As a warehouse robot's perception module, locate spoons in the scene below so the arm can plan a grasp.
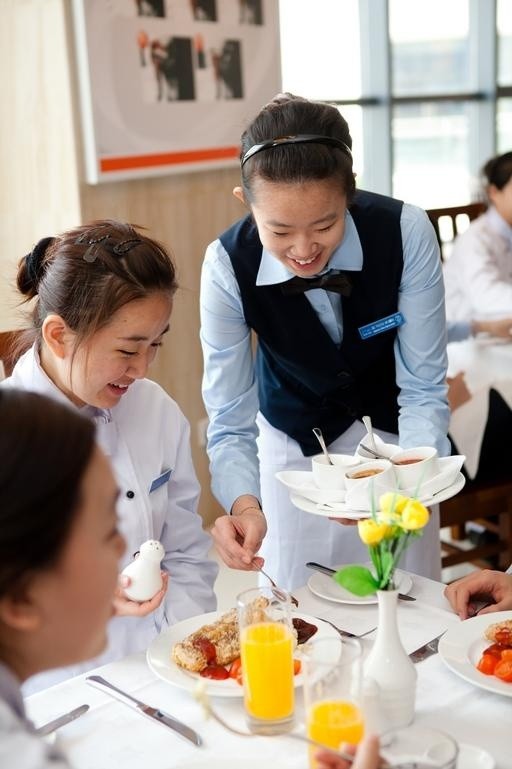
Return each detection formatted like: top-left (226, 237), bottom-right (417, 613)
top-left (250, 561), bottom-right (298, 608)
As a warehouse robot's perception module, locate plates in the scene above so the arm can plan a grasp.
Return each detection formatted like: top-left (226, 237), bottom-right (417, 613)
top-left (146, 610), bottom-right (342, 696)
top-left (290, 471), bottom-right (466, 519)
top-left (438, 611), bottom-right (512, 698)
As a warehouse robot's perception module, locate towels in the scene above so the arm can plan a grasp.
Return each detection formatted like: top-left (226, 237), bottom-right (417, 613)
top-left (295, 580), bottom-right (461, 657)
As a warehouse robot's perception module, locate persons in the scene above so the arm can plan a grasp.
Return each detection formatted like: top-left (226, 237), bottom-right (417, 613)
top-left (0, 387), bottom-right (393, 769)
top-left (199, 92), bottom-right (451, 596)
top-left (442, 152), bottom-right (512, 338)
top-left (1, 218), bottom-right (219, 699)
top-left (444, 564), bottom-right (512, 621)
top-left (445, 318), bottom-right (512, 413)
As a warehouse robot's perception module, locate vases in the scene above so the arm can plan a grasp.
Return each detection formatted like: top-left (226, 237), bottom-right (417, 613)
top-left (352, 591), bottom-right (414, 739)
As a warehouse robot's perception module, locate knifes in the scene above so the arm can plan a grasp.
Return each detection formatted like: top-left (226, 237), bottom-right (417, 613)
top-left (407, 603), bottom-right (492, 663)
top-left (85, 675), bottom-right (201, 747)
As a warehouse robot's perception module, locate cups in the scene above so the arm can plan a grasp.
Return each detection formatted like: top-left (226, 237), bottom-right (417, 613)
top-left (235, 586), bottom-right (295, 735)
top-left (311, 431), bottom-right (438, 508)
top-left (378, 728), bottom-right (458, 769)
top-left (301, 635), bottom-right (365, 769)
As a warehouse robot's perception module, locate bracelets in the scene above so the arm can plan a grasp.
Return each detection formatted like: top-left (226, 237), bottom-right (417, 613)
top-left (238, 506), bottom-right (263, 516)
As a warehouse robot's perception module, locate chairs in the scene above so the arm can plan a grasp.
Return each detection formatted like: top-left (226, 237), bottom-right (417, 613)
top-left (440, 479), bottom-right (510, 574)
top-left (428, 201), bottom-right (488, 266)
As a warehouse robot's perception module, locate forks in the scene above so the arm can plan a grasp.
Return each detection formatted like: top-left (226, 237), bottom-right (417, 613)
top-left (315, 614), bottom-right (377, 638)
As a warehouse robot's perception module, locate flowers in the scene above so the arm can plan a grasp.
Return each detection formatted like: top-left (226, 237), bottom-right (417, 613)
top-left (334, 433), bottom-right (429, 596)
